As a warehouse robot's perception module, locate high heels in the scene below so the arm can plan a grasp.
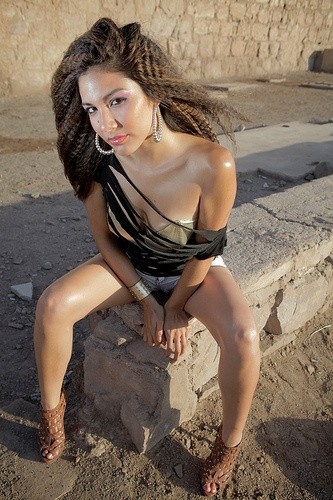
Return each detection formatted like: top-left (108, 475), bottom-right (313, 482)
top-left (37, 393), bottom-right (67, 463)
top-left (200, 422), bottom-right (242, 497)
